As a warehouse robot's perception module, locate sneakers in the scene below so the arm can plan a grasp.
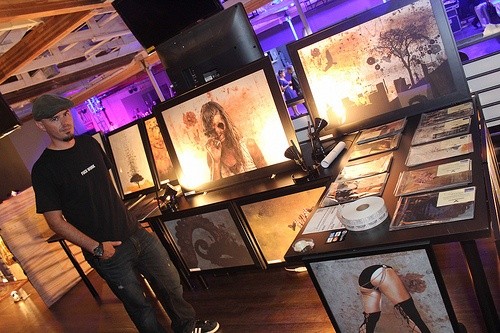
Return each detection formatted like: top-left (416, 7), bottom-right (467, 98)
top-left (189, 320), bottom-right (220, 333)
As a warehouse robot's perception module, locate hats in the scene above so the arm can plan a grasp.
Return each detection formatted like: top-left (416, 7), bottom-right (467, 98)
top-left (31, 95), bottom-right (73, 121)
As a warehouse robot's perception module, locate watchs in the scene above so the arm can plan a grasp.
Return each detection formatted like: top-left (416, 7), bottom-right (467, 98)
top-left (93, 242), bottom-right (104, 257)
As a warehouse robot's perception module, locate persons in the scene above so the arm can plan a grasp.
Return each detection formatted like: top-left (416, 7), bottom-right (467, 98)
top-left (201, 101), bottom-right (267, 182)
top-left (278, 70), bottom-right (300, 115)
top-left (358, 264), bottom-right (431, 333)
top-left (287, 65), bottom-right (308, 111)
top-left (31, 94), bottom-right (220, 333)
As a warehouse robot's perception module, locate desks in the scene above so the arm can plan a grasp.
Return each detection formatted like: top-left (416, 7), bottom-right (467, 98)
top-left (45, 93), bottom-right (500, 333)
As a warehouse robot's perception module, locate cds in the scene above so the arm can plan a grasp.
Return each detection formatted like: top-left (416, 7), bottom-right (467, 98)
top-left (338, 197), bottom-right (388, 231)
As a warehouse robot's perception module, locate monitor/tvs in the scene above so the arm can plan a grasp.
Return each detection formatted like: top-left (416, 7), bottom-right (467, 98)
top-left (156, 1), bottom-right (265, 94)
top-left (150, 54), bottom-right (304, 197)
top-left (285, 0), bottom-right (473, 139)
top-left (0, 91), bottom-right (23, 139)
top-left (102, 119), bottom-right (161, 200)
top-left (110, 0), bottom-right (224, 54)
